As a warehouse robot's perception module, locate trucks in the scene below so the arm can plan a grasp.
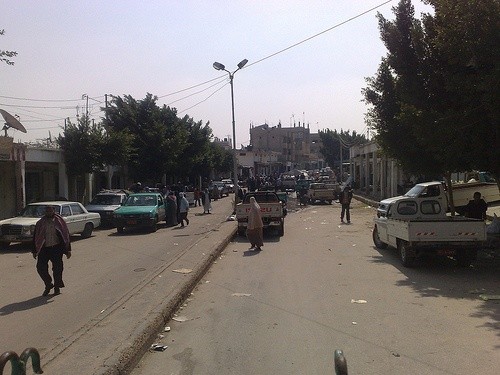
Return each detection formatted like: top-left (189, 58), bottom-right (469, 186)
top-left (296, 179), bottom-right (315, 198)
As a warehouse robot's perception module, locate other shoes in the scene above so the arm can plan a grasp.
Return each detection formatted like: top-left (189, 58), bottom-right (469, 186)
top-left (180, 224), bottom-right (184, 228)
top-left (347, 219), bottom-right (350, 223)
top-left (187, 219), bottom-right (189, 225)
top-left (341, 218), bottom-right (344, 222)
top-left (53, 288), bottom-right (60, 296)
top-left (42, 282), bottom-right (53, 296)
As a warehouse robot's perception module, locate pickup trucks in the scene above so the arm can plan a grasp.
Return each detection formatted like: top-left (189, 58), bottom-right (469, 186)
top-left (206, 181), bottom-right (230, 199)
top-left (305, 183), bottom-right (333, 204)
top-left (373, 196), bottom-right (487, 269)
top-left (114, 193), bottom-right (166, 233)
top-left (377, 180), bottom-right (500, 219)
top-left (235, 192), bottom-right (285, 237)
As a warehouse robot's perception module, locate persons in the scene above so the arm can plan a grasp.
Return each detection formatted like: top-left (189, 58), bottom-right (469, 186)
top-left (464, 192), bottom-right (487, 219)
top-left (128, 172), bottom-right (353, 228)
top-left (247, 197), bottom-right (264, 252)
top-left (32, 205), bottom-right (71, 296)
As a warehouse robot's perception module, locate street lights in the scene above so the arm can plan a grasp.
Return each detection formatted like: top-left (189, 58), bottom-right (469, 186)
top-left (213, 59), bottom-right (248, 213)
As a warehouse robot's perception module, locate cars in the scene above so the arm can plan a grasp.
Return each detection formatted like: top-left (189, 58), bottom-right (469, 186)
top-left (279, 166), bottom-right (337, 196)
top-left (83, 192), bottom-right (126, 227)
top-left (221, 179), bottom-right (235, 194)
top-left (0, 201), bottom-right (101, 251)
